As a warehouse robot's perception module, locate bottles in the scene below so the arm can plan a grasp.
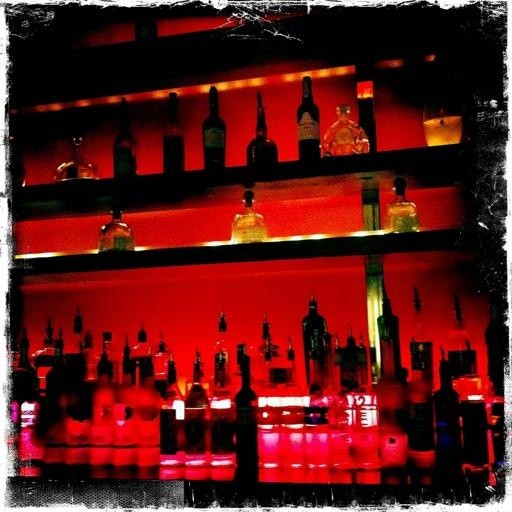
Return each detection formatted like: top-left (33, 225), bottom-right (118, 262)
top-left (55, 133), bottom-right (94, 181)
top-left (112, 98), bottom-right (138, 177)
top-left (17, 292), bottom-right (487, 469)
top-left (383, 177), bottom-right (419, 232)
top-left (201, 87), bottom-right (226, 166)
top-left (100, 202), bottom-right (132, 255)
top-left (294, 77), bottom-right (324, 160)
top-left (320, 104), bottom-right (371, 154)
top-left (246, 93), bottom-right (279, 165)
top-left (162, 91), bottom-right (184, 171)
top-left (231, 181), bottom-right (268, 243)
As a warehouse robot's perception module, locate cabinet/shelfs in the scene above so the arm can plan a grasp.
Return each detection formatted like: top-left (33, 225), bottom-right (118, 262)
top-left (12, 143), bottom-right (466, 274)
